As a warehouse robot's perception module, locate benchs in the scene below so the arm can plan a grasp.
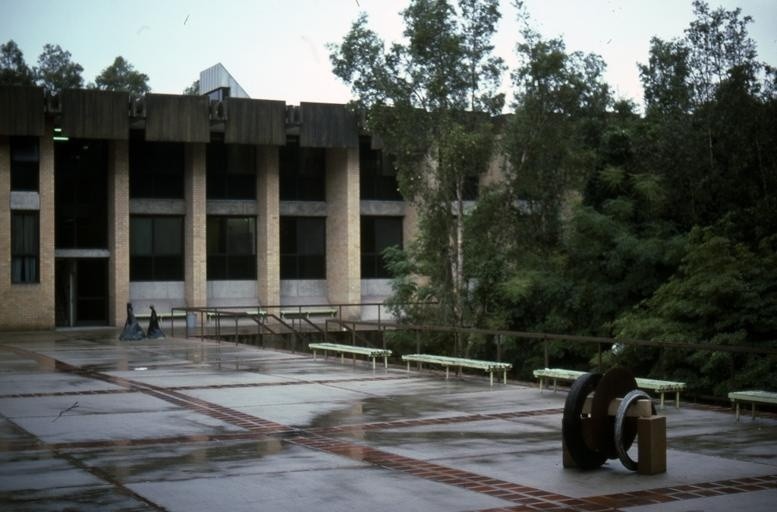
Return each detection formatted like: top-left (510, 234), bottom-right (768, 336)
top-left (727, 389), bottom-right (776, 420)
top-left (401, 352), bottom-right (513, 385)
top-left (133, 311), bottom-right (186, 325)
top-left (533, 367), bottom-right (687, 408)
top-left (309, 342), bottom-right (394, 370)
top-left (206, 309), bottom-right (338, 325)
